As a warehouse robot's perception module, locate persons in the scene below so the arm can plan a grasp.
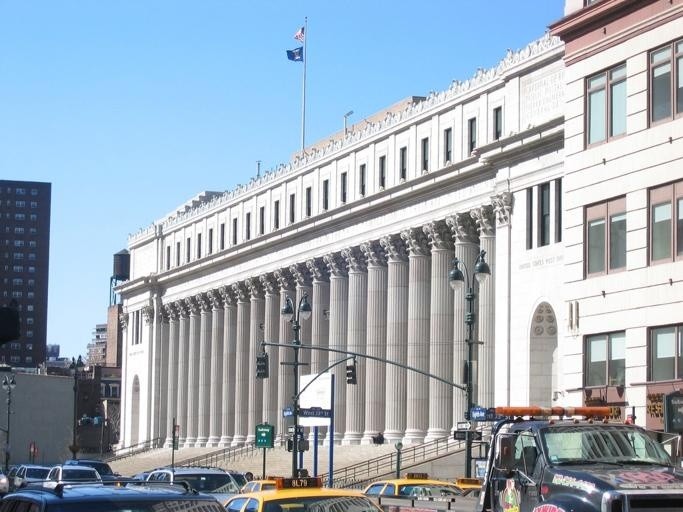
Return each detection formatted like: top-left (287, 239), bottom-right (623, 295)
top-left (245, 472), bottom-right (253, 481)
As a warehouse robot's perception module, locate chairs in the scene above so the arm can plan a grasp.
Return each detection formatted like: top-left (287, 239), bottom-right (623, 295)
top-left (517, 445), bottom-right (540, 476)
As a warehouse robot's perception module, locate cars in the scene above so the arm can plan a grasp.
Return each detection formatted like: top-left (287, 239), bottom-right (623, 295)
top-left (0, 458), bottom-right (485, 511)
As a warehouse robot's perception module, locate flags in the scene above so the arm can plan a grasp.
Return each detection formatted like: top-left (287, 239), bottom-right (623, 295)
top-left (286, 25), bottom-right (304, 63)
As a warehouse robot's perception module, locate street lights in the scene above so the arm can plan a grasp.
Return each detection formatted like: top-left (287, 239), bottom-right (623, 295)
top-left (0, 375), bottom-right (16, 467)
top-left (447, 251), bottom-right (490, 479)
top-left (279, 293), bottom-right (312, 480)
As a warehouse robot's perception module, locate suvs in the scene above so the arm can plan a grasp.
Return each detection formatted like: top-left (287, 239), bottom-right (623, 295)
top-left (478, 404), bottom-right (683, 511)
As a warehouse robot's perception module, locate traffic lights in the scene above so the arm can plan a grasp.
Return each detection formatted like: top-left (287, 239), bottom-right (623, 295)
top-left (255, 354), bottom-right (268, 378)
top-left (344, 365), bottom-right (355, 383)
